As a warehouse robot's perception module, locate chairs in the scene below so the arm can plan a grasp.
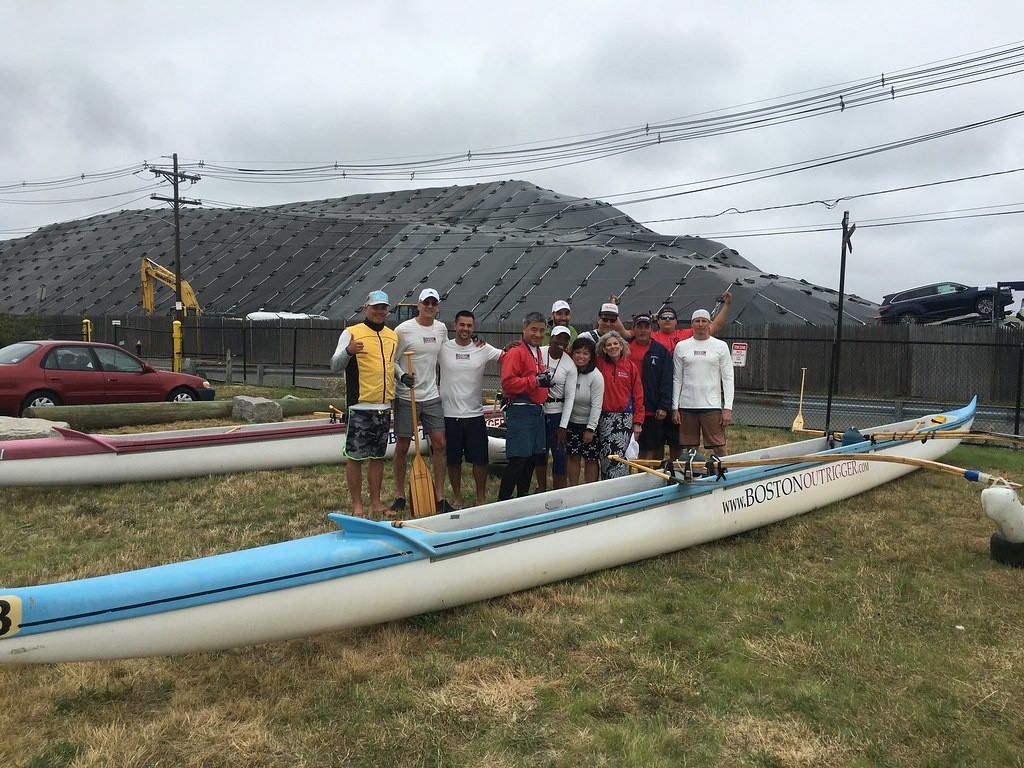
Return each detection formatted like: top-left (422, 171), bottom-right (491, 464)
top-left (59, 353), bottom-right (77, 369)
top-left (74, 356), bottom-right (92, 370)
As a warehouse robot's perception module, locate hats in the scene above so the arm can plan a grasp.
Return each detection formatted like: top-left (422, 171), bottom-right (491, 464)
top-left (691, 309), bottom-right (712, 326)
top-left (625, 432), bottom-right (639, 461)
top-left (598, 303), bottom-right (619, 316)
top-left (364, 291), bottom-right (391, 308)
top-left (658, 306), bottom-right (677, 328)
top-left (418, 288), bottom-right (440, 302)
top-left (632, 316), bottom-right (652, 328)
top-left (551, 325), bottom-right (571, 338)
top-left (550, 300), bottom-right (571, 313)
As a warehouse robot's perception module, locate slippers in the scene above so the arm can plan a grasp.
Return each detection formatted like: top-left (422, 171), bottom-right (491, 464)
top-left (453, 499), bottom-right (464, 509)
top-left (368, 507), bottom-right (396, 517)
top-left (352, 509), bottom-right (369, 520)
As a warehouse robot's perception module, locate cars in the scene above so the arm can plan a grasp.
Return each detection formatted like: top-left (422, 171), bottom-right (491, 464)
top-left (0, 340), bottom-right (216, 419)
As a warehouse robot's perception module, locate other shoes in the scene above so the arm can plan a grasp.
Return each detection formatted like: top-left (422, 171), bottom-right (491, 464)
top-left (436, 498), bottom-right (455, 513)
top-left (391, 497), bottom-right (406, 512)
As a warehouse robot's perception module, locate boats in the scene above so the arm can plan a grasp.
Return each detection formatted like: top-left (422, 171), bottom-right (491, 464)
top-left (0, 405), bottom-right (520, 487)
top-left (0, 395), bottom-right (979, 667)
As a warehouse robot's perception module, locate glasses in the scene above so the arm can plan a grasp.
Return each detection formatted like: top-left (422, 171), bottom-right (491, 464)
top-left (660, 314), bottom-right (675, 320)
top-left (419, 300), bottom-right (438, 306)
top-left (601, 318), bottom-right (615, 322)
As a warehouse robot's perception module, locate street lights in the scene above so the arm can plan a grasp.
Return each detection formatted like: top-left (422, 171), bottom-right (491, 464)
top-left (134, 214), bottom-right (182, 357)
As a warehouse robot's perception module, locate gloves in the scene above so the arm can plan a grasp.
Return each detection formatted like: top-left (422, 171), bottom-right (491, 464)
top-left (535, 369), bottom-right (551, 387)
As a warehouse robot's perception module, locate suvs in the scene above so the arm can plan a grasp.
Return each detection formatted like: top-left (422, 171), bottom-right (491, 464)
top-left (879, 282), bottom-right (1014, 326)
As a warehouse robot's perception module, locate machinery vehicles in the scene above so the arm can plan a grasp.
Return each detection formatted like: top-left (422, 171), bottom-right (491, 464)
top-left (140, 253), bottom-right (202, 317)
top-left (346, 303), bottom-right (441, 321)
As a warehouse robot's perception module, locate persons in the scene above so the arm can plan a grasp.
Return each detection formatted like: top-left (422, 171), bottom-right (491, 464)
top-left (504, 292), bottom-right (735, 490)
top-left (330, 290), bottom-right (415, 521)
top-left (437, 310), bottom-right (504, 510)
top-left (391, 288), bottom-right (486, 513)
top-left (497, 312), bottom-right (557, 503)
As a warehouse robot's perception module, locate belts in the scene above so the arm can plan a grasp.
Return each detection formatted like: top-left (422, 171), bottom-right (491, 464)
top-left (512, 398), bottom-right (533, 403)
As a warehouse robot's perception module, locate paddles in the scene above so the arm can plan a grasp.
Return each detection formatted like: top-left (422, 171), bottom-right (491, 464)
top-left (794, 429), bottom-right (1024, 444)
top-left (403, 351), bottom-right (437, 518)
top-left (608, 454), bottom-right (686, 485)
top-left (912, 420), bottom-right (925, 430)
top-left (630, 453), bottom-right (1024, 491)
top-left (792, 368), bottom-right (807, 430)
top-left (225, 426), bottom-right (241, 433)
